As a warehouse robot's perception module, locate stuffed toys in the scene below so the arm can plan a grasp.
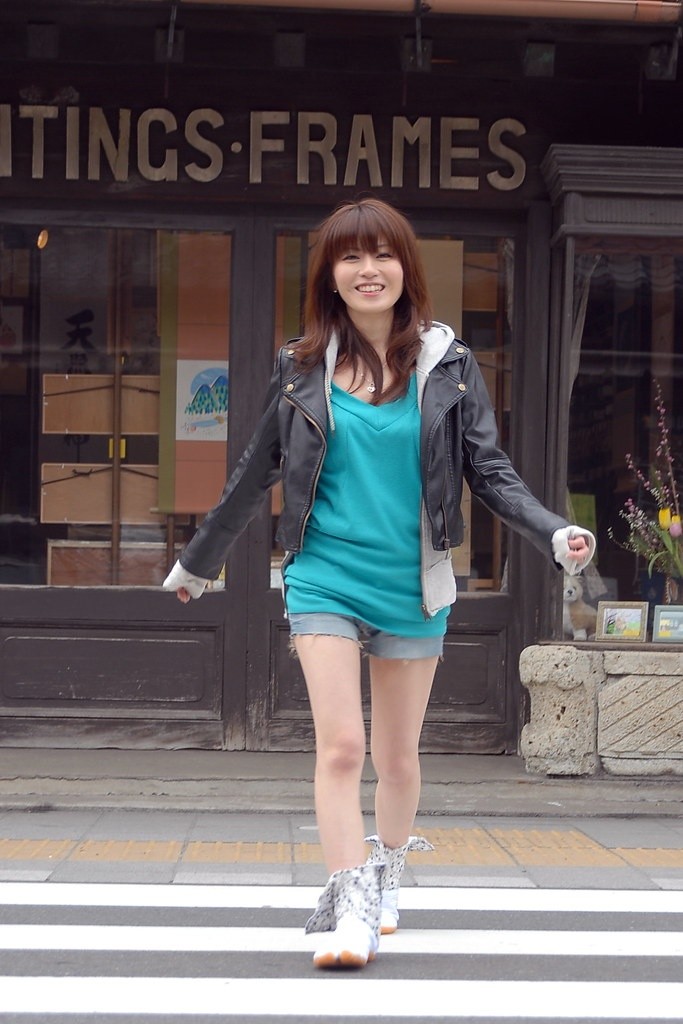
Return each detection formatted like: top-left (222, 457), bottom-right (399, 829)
top-left (564, 574), bottom-right (597, 640)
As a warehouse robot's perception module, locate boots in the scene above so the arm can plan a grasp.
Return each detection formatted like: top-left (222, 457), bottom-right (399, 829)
top-left (305, 861), bottom-right (386, 969)
top-left (364, 832), bottom-right (435, 936)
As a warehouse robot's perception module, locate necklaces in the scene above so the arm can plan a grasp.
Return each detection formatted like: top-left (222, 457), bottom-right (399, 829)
top-left (343, 351), bottom-right (389, 394)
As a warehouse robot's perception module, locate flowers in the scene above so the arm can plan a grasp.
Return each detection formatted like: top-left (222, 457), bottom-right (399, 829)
top-left (607, 375), bottom-right (683, 605)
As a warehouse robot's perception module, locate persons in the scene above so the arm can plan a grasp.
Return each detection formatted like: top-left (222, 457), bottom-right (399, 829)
top-left (161, 199), bottom-right (599, 967)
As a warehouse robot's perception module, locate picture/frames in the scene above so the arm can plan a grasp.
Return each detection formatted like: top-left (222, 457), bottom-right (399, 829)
top-left (652, 605), bottom-right (683, 644)
top-left (596, 601), bottom-right (649, 643)
